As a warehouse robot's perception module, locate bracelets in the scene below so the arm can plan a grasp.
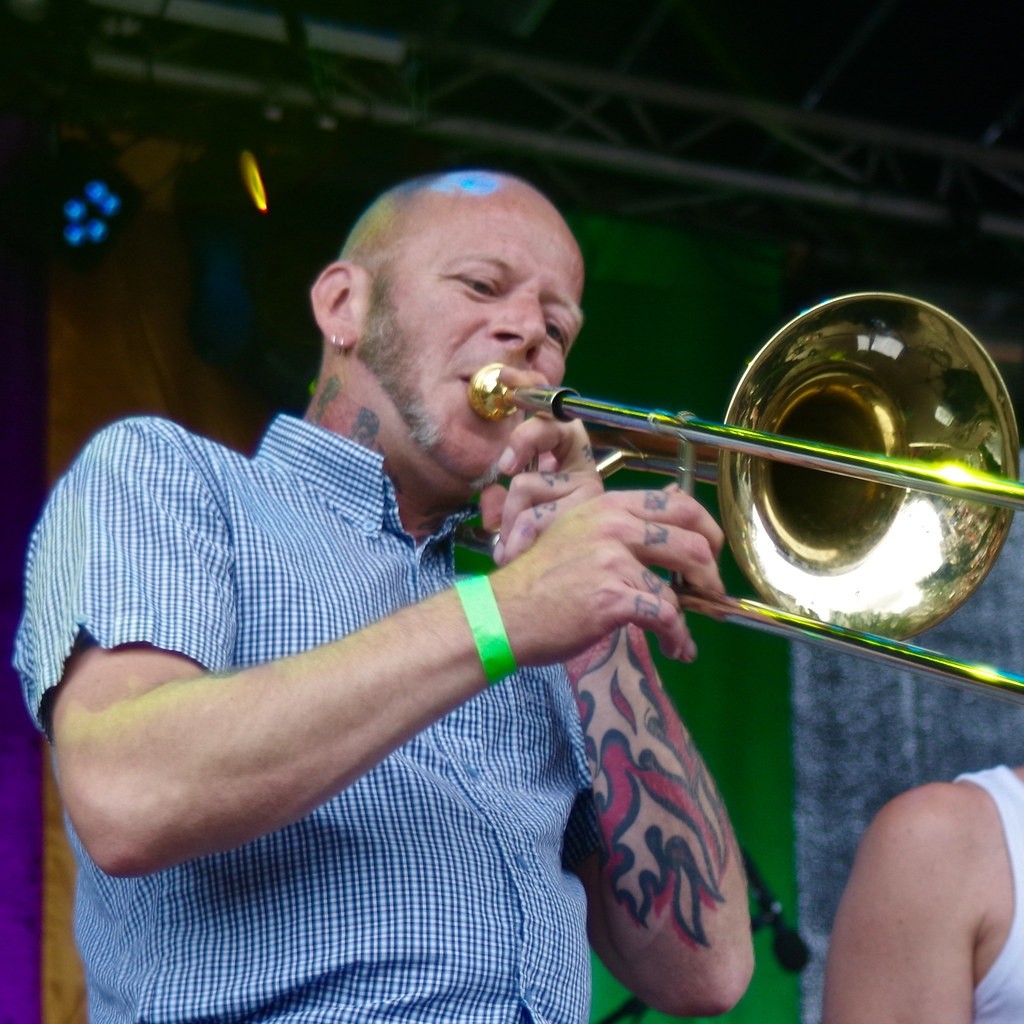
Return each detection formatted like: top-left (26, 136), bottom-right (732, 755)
top-left (453, 572), bottom-right (516, 683)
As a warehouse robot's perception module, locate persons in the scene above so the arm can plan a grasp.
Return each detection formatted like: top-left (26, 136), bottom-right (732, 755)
top-left (14, 172), bottom-right (755, 1024)
top-left (822, 764), bottom-right (1024, 1023)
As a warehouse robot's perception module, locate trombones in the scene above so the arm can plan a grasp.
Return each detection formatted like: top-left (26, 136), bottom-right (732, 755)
top-left (467, 288), bottom-right (1024, 695)
